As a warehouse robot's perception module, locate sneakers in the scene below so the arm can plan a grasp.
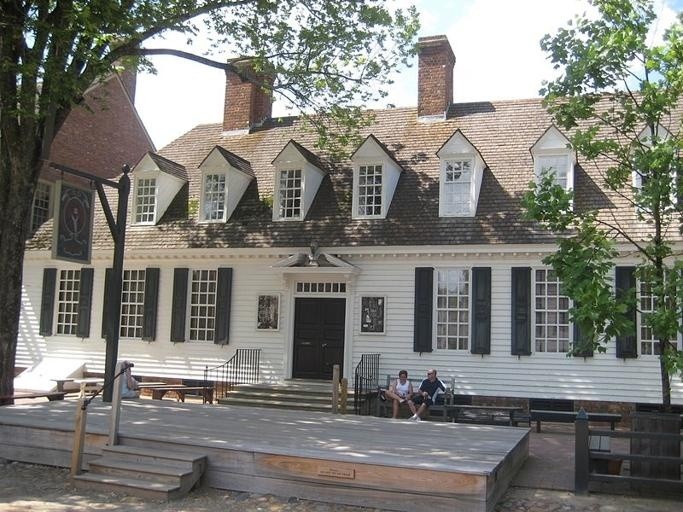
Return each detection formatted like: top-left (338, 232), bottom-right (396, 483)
top-left (408, 417), bottom-right (421, 421)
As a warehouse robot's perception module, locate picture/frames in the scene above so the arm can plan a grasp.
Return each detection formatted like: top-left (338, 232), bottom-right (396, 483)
top-left (255, 294), bottom-right (279, 332)
top-left (361, 295), bottom-right (386, 336)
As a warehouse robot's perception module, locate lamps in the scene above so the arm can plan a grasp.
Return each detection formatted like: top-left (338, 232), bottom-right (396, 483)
top-left (308, 240), bottom-right (321, 265)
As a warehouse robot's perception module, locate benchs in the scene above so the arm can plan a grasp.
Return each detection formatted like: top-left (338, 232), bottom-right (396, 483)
top-left (376, 374), bottom-right (622, 472)
top-left (0, 378), bottom-right (216, 404)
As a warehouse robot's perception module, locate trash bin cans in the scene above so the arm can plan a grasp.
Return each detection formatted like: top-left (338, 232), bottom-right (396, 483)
top-left (589, 449), bottom-right (623, 475)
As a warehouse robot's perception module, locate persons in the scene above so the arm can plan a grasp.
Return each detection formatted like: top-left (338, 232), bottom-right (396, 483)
top-left (385, 370), bottom-right (414, 418)
top-left (122, 361), bottom-right (141, 399)
top-left (408, 369), bottom-right (448, 420)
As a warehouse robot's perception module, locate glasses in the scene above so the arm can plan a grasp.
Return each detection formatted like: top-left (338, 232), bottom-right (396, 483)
top-left (427, 373), bottom-right (433, 375)
